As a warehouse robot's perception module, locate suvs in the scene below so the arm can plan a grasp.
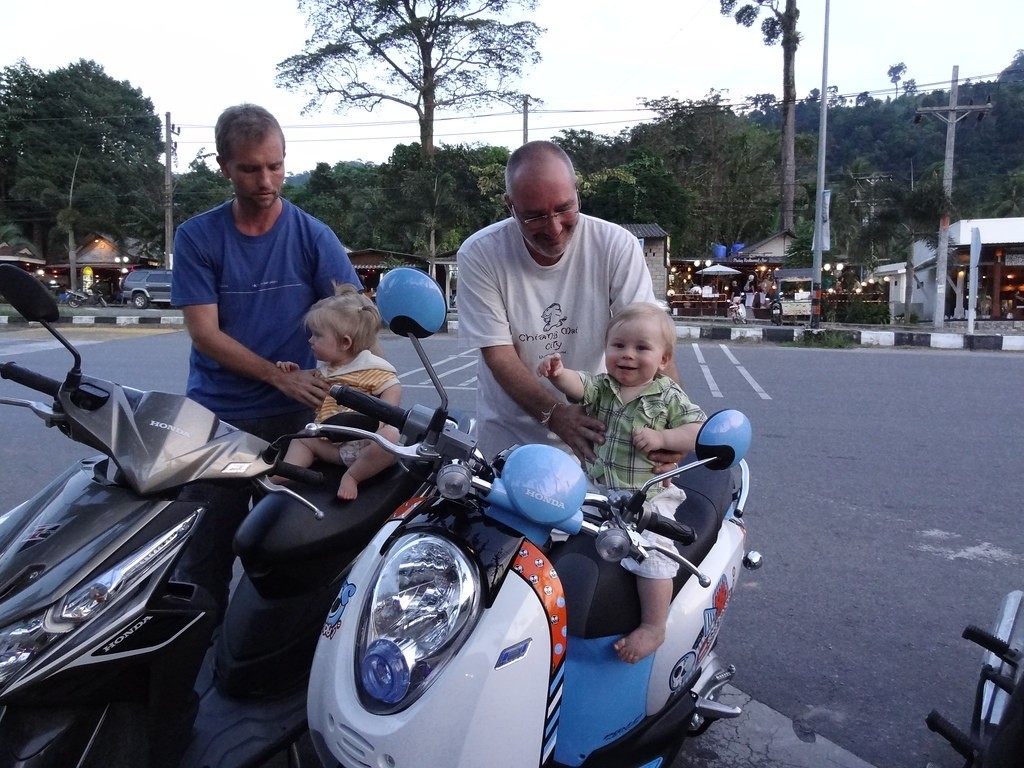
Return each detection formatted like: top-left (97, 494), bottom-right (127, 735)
top-left (120, 268), bottom-right (173, 309)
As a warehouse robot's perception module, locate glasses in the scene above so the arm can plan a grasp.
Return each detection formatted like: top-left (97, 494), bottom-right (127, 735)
top-left (512, 191), bottom-right (581, 226)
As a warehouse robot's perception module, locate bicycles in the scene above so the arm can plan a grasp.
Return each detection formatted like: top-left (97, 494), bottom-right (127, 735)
top-left (729, 303), bottom-right (747, 325)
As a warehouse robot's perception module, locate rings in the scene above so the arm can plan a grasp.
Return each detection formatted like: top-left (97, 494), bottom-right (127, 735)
top-left (674, 463), bottom-right (679, 468)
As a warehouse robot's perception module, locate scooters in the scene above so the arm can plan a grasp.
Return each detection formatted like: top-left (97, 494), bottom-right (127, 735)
top-left (0, 258), bottom-right (456, 768)
top-left (282, 263), bottom-right (763, 767)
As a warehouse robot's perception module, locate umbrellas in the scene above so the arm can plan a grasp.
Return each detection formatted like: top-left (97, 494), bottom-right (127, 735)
top-left (695, 263), bottom-right (742, 293)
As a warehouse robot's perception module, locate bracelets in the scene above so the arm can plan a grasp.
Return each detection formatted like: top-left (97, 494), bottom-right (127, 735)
top-left (540, 402), bottom-right (565, 432)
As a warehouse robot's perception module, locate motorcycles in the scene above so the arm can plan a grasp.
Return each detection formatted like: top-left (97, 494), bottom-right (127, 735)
top-left (764, 287), bottom-right (784, 326)
top-left (64, 274), bottom-right (109, 309)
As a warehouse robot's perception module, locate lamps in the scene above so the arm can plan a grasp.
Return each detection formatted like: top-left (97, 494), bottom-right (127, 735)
top-left (995, 248), bottom-right (1002, 263)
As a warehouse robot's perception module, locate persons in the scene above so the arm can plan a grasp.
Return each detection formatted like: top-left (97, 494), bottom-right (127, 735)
top-left (170, 102), bottom-right (385, 618)
top-left (270, 282), bottom-right (402, 499)
top-left (836, 275), bottom-right (861, 293)
top-left (456, 141), bottom-right (678, 487)
top-left (746, 275), bottom-right (755, 295)
top-left (535, 303), bottom-right (708, 664)
top-left (732, 280), bottom-right (742, 296)
